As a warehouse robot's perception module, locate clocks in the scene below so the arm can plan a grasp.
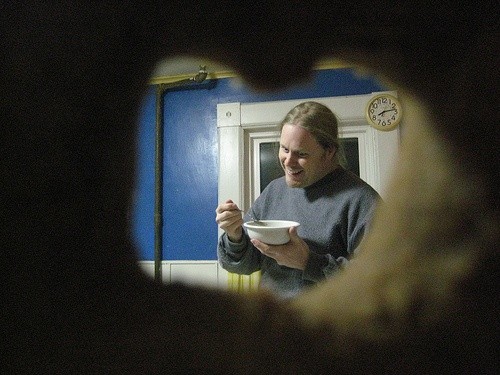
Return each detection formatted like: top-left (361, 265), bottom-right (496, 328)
top-left (365, 94), bottom-right (403, 131)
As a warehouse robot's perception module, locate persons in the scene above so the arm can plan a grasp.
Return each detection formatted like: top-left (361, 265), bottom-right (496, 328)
top-left (215, 101), bottom-right (385, 298)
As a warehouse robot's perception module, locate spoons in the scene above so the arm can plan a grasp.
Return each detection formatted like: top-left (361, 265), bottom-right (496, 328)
top-left (239, 210), bottom-right (268, 226)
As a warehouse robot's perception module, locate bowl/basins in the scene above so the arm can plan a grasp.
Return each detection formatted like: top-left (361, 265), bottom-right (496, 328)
top-left (243, 220), bottom-right (300, 245)
